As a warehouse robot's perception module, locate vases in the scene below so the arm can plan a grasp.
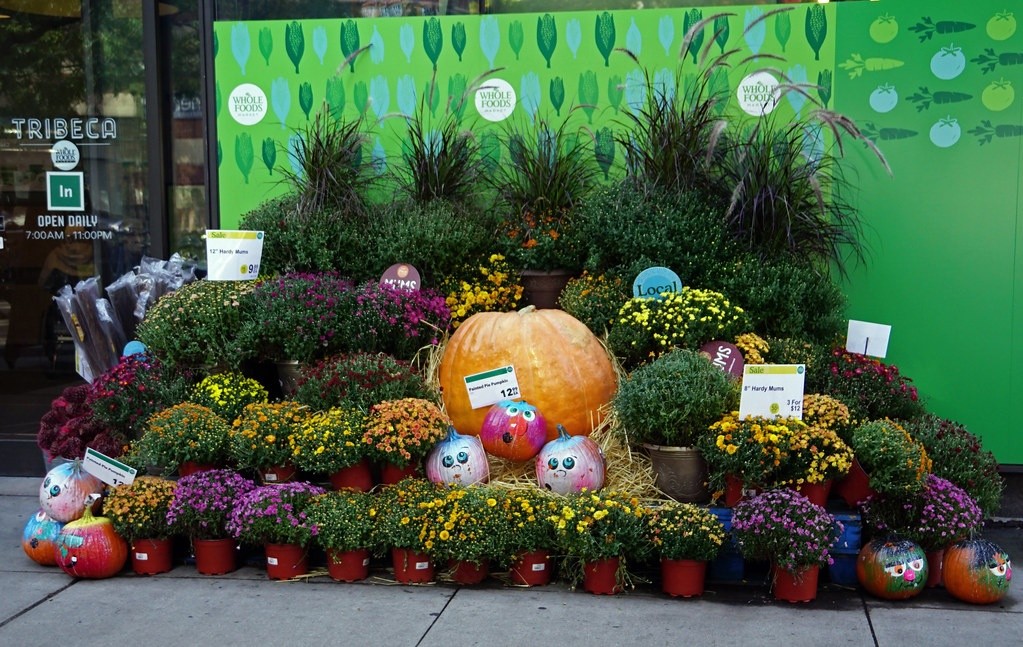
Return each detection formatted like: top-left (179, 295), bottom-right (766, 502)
top-left (380, 460), bottom-right (419, 487)
top-left (799, 477), bottom-right (834, 507)
top-left (660, 556), bottom-right (706, 598)
top-left (643, 443), bottom-right (706, 503)
top-left (584, 556), bottom-right (624, 596)
top-left (180, 460), bottom-right (217, 476)
top-left (838, 456), bottom-right (870, 508)
top-left (445, 556), bottom-right (486, 584)
top-left (724, 474), bottom-right (762, 508)
top-left (130, 537), bottom-right (172, 574)
top-left (327, 546), bottom-right (370, 583)
top-left (509, 549), bottom-right (553, 584)
top-left (773, 563), bottom-right (816, 603)
top-left (518, 269), bottom-right (567, 308)
top-left (264, 540), bottom-right (306, 579)
top-left (191, 536), bottom-right (239, 574)
top-left (394, 548), bottom-right (435, 584)
top-left (925, 549), bottom-right (946, 587)
top-left (273, 359), bottom-right (313, 396)
top-left (263, 462), bottom-right (295, 484)
top-left (330, 459), bottom-right (371, 492)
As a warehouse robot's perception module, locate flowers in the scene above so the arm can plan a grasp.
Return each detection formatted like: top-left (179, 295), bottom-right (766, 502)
top-left (38, 7), bottom-right (1004, 593)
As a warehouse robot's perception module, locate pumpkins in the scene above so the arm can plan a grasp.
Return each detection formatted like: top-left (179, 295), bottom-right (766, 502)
top-left (855, 530), bottom-right (1012, 603)
top-left (437, 304), bottom-right (620, 444)
top-left (425, 399), bottom-right (604, 497)
top-left (23, 455), bottom-right (129, 577)
